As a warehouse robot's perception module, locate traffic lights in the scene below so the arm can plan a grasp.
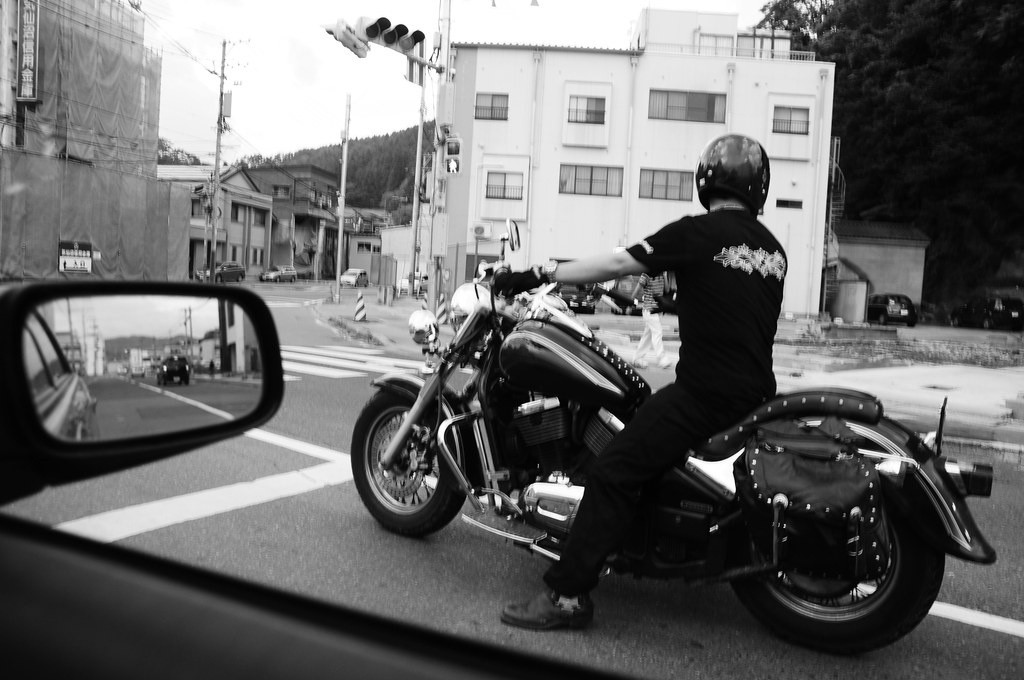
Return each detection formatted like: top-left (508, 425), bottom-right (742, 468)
top-left (443, 138), bottom-right (460, 174)
top-left (353, 13), bottom-right (426, 57)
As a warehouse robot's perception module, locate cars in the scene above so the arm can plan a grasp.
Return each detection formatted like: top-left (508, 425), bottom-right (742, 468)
top-left (156, 355), bottom-right (192, 385)
top-left (259, 264), bottom-right (298, 283)
top-left (868, 291), bottom-right (918, 326)
top-left (948, 293), bottom-right (1024, 331)
top-left (339, 268), bottom-right (368, 288)
top-left (547, 257), bottom-right (599, 314)
top-left (611, 271), bottom-right (663, 316)
top-left (117, 368), bottom-right (130, 377)
top-left (194, 261), bottom-right (246, 282)
top-left (132, 363), bottom-right (146, 378)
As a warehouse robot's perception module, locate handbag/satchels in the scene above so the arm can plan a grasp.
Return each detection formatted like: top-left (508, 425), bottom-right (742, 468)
top-left (654, 292), bottom-right (673, 310)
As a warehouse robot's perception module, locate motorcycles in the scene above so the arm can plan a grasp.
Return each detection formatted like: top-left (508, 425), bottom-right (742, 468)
top-left (348, 214), bottom-right (998, 662)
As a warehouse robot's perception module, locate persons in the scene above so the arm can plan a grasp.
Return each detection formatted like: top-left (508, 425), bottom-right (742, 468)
top-left (495, 133), bottom-right (788, 630)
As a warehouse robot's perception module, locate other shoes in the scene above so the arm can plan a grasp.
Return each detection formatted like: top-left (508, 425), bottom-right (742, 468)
top-left (656, 360), bottom-right (672, 368)
top-left (632, 361), bottom-right (649, 368)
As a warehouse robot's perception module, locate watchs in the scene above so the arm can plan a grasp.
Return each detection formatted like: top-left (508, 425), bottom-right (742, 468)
top-left (545, 260), bottom-right (558, 283)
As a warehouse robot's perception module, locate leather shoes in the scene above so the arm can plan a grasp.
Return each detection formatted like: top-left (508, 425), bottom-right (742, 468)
top-left (498, 586), bottom-right (594, 629)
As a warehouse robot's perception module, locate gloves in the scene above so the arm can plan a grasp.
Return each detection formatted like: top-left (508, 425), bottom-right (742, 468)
top-left (493, 265), bottom-right (551, 305)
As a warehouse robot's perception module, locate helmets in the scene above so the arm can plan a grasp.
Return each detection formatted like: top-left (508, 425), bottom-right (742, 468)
top-left (695, 134), bottom-right (771, 212)
